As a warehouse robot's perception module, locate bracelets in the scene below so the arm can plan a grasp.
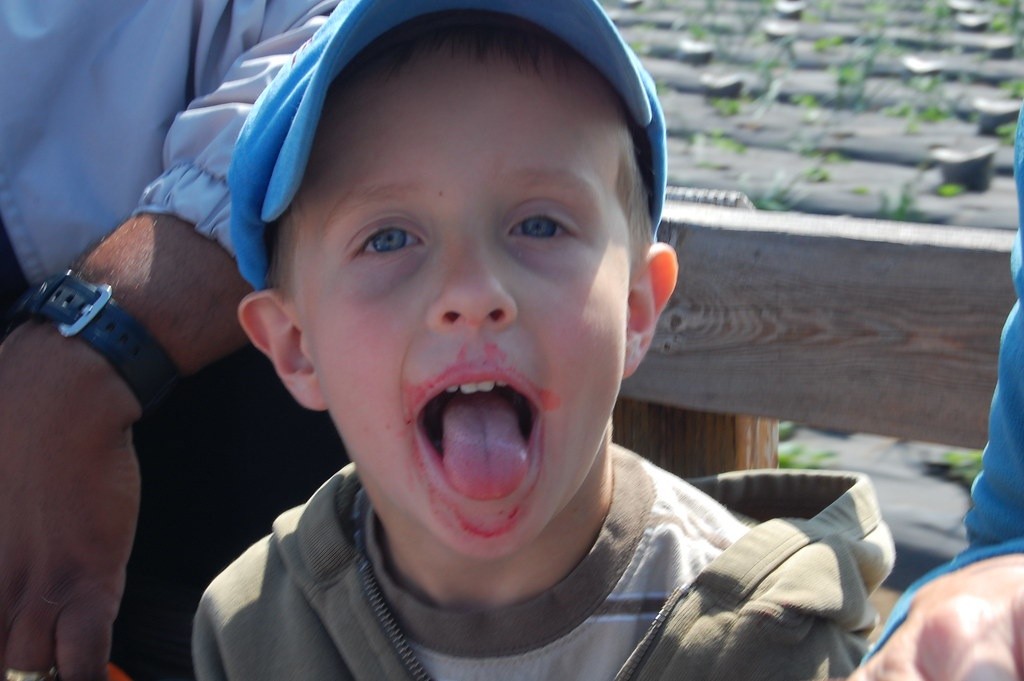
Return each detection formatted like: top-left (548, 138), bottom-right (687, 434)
top-left (12, 270), bottom-right (180, 420)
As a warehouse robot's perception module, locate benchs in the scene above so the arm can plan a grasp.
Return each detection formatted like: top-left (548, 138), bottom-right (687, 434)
top-left (610, 175), bottom-right (1017, 552)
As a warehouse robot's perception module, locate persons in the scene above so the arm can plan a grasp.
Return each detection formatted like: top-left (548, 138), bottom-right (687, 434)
top-left (0, 0), bottom-right (343, 681)
top-left (191, 0), bottom-right (898, 681)
top-left (852, 103), bottom-right (1024, 681)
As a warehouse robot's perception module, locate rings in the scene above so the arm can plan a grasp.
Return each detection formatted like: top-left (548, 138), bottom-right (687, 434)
top-left (4, 663), bottom-right (59, 681)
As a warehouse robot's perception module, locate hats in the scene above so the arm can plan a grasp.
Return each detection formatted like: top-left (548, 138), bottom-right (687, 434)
top-left (229, 0), bottom-right (667, 293)
